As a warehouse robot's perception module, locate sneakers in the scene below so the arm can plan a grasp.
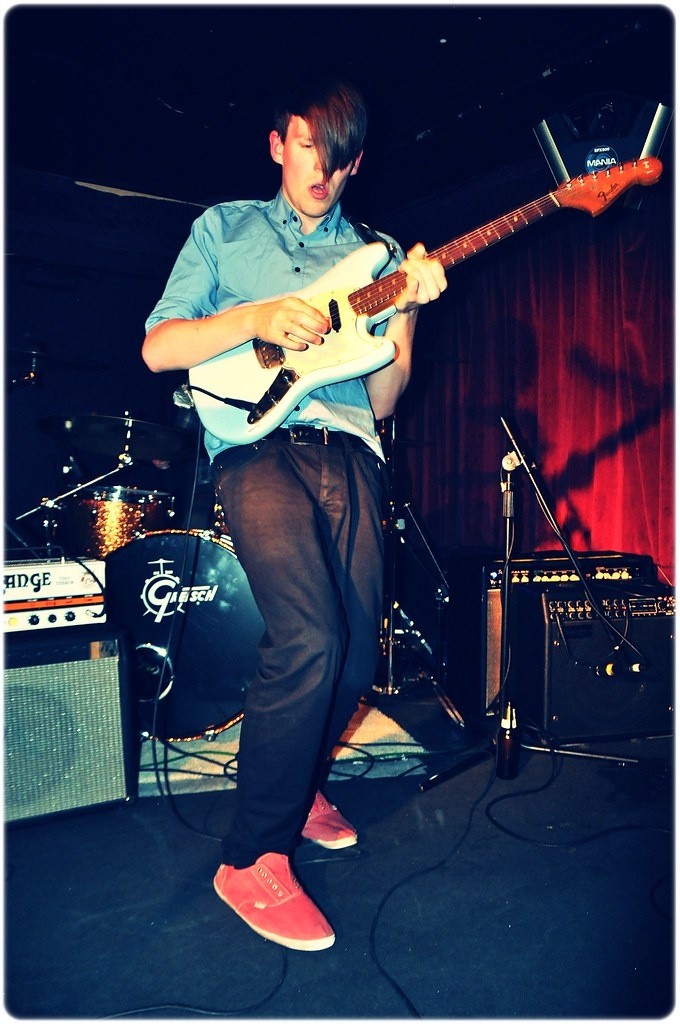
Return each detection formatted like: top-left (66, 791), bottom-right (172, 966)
top-left (301, 789), bottom-right (357, 849)
top-left (214, 853), bottom-right (335, 951)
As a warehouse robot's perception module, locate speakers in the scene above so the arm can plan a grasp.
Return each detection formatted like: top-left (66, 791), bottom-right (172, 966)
top-left (4, 622), bottom-right (136, 822)
top-left (505, 584), bottom-right (675, 748)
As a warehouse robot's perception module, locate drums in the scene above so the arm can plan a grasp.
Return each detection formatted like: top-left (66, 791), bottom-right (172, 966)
top-left (102, 529), bottom-right (266, 740)
top-left (54, 484), bottom-right (175, 560)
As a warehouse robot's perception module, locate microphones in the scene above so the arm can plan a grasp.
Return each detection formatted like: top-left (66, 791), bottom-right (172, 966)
top-left (595, 663), bottom-right (645, 677)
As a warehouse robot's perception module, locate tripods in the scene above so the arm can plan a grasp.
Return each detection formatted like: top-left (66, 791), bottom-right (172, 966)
top-left (418, 415), bottom-right (639, 794)
top-left (372, 414), bottom-right (467, 730)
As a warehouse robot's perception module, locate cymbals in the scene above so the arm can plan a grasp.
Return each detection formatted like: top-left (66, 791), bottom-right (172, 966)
top-left (7, 346), bottom-right (107, 368)
top-left (36, 413), bottom-right (175, 432)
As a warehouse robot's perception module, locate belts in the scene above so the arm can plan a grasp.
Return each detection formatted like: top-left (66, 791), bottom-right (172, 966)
top-left (266, 425), bottom-right (368, 447)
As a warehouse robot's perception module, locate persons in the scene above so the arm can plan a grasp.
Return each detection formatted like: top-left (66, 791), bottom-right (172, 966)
top-left (141, 77), bottom-right (448, 952)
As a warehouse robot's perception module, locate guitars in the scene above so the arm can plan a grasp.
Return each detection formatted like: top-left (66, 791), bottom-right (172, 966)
top-left (187, 156), bottom-right (662, 445)
